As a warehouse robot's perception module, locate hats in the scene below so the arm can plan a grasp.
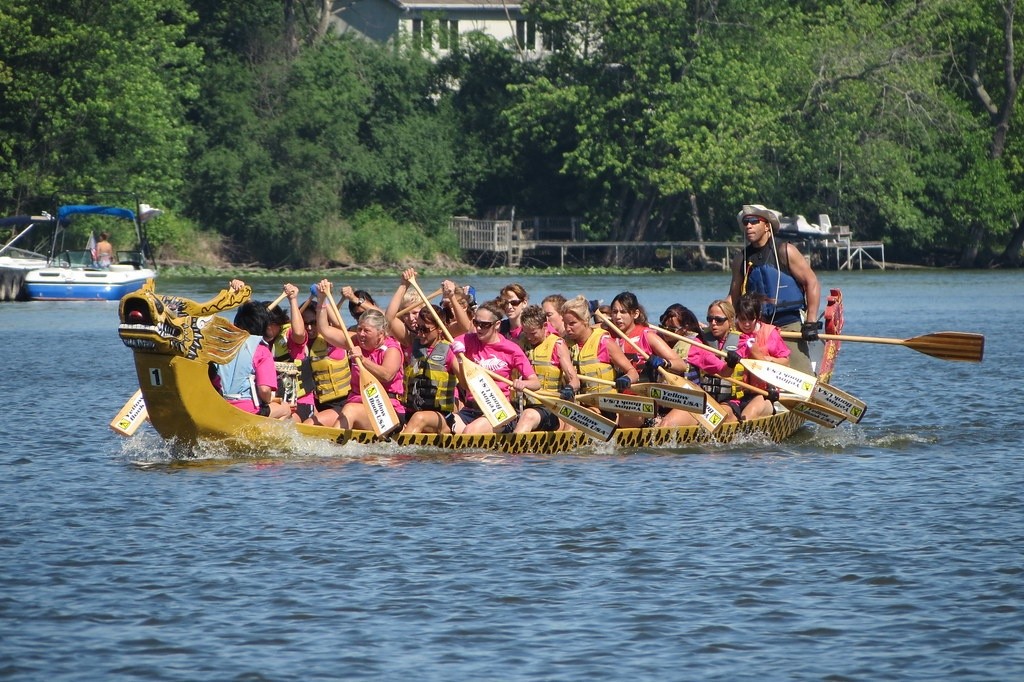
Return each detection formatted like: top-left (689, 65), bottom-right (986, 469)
top-left (736, 205), bottom-right (781, 235)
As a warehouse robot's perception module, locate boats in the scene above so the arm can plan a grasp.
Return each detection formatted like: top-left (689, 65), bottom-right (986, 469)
top-left (21, 189), bottom-right (159, 302)
top-left (0, 214), bottom-right (63, 300)
top-left (118, 279), bottom-right (844, 450)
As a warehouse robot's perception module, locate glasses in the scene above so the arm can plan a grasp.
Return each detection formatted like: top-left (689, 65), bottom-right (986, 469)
top-left (502, 299), bottom-right (523, 306)
top-left (414, 324), bottom-right (438, 333)
top-left (658, 323), bottom-right (684, 334)
top-left (742, 216), bottom-right (760, 226)
top-left (472, 318), bottom-right (498, 329)
top-left (706, 316), bottom-right (731, 323)
top-left (303, 318), bottom-right (316, 326)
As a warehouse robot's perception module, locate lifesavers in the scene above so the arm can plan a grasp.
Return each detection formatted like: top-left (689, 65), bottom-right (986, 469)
top-left (0, 271), bottom-right (25, 301)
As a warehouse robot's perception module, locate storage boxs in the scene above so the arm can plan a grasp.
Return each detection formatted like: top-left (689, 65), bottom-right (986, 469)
top-left (834, 225), bottom-right (851, 235)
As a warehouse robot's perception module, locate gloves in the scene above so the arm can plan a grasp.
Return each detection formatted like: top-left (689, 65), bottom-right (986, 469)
top-left (612, 374), bottom-right (631, 393)
top-left (647, 353), bottom-right (668, 369)
top-left (725, 350), bottom-right (741, 368)
top-left (449, 341), bottom-right (465, 354)
top-left (763, 385), bottom-right (779, 403)
top-left (800, 321), bottom-right (819, 341)
top-left (559, 384), bottom-right (575, 401)
top-left (588, 300), bottom-right (600, 317)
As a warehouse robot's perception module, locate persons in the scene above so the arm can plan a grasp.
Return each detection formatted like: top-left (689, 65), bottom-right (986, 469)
top-left (207, 267), bottom-right (790, 436)
top-left (96, 232), bottom-right (113, 268)
top-left (726, 204), bottom-right (823, 379)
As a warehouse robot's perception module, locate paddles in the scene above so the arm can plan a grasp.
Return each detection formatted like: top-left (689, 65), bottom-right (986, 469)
top-left (714, 372), bottom-right (848, 430)
top-left (561, 370), bottom-right (708, 415)
top-left (486, 368), bottom-right (618, 443)
top-left (646, 322), bottom-right (818, 399)
top-left (407, 273), bottom-right (519, 430)
top-left (335, 295), bottom-right (346, 310)
top-left (533, 388), bottom-right (658, 420)
top-left (701, 323), bottom-right (985, 364)
top-left (595, 307), bottom-right (728, 435)
top-left (298, 291), bottom-right (316, 313)
top-left (396, 282), bottom-right (449, 318)
top-left (321, 282), bottom-right (401, 441)
top-left (812, 380), bottom-right (869, 425)
top-left (108, 284), bottom-right (294, 438)
